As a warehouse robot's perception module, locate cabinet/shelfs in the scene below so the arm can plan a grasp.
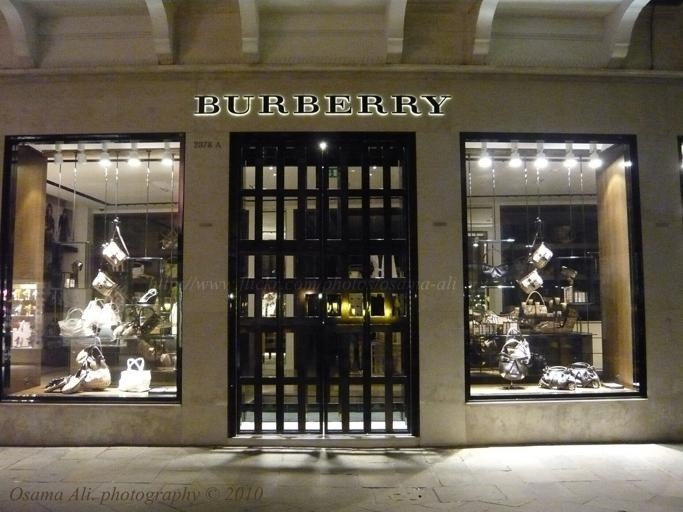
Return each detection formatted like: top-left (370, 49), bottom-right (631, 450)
top-left (10, 279), bottom-right (42, 393)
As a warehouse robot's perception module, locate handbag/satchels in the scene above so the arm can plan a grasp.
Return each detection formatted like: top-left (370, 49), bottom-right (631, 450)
top-left (479, 291), bottom-right (576, 336)
top-left (90, 271), bottom-right (116, 298)
top-left (43, 336), bottom-right (151, 393)
top-left (102, 224), bottom-right (130, 268)
top-left (497, 328), bottom-right (600, 390)
top-left (131, 262), bottom-right (144, 279)
top-left (480, 263), bottom-right (509, 286)
top-left (161, 232), bottom-right (177, 251)
top-left (515, 268), bottom-right (544, 295)
top-left (527, 232), bottom-right (553, 270)
top-left (56, 297), bottom-right (159, 336)
top-left (164, 257), bottom-right (177, 278)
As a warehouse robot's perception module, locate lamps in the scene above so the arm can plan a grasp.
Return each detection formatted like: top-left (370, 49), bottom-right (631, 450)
top-left (54, 139), bottom-right (173, 166)
top-left (477, 139), bottom-right (603, 169)
